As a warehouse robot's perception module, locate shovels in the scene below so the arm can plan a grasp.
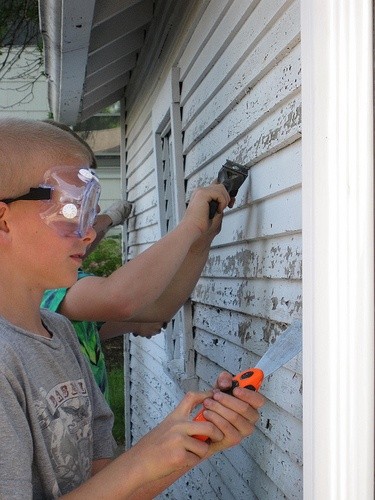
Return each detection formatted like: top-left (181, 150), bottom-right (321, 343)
top-left (185, 316), bottom-right (302, 447)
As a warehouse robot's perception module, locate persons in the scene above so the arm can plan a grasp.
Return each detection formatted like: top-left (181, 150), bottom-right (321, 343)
top-left (38, 119), bottom-right (231, 408)
top-left (84, 201), bottom-right (132, 258)
top-left (0, 112), bottom-right (267, 500)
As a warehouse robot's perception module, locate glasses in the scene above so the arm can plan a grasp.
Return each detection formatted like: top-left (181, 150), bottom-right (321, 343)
top-left (41, 166), bottom-right (102, 240)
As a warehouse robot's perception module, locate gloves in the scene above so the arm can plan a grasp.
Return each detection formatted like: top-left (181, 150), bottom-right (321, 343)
top-left (103, 200), bottom-right (133, 230)
